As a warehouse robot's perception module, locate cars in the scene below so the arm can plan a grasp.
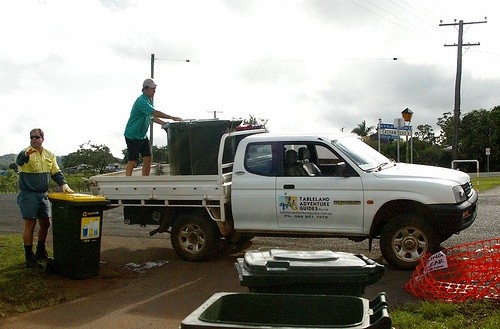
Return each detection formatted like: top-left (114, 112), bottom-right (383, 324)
top-left (0, 170), bottom-right (7, 176)
top-left (68, 164), bottom-right (88, 173)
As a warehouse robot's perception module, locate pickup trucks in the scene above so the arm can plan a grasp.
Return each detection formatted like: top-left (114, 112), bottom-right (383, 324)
top-left (89, 125), bottom-right (478, 272)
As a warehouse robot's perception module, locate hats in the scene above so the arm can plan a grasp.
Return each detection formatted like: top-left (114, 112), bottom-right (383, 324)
top-left (143, 79), bottom-right (157, 87)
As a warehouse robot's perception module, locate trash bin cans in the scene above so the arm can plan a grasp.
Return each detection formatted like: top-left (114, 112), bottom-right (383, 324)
top-left (235, 247), bottom-right (385, 296)
top-left (162, 119), bottom-right (243, 175)
top-left (47, 193), bottom-right (110, 280)
top-left (181, 291), bottom-right (393, 329)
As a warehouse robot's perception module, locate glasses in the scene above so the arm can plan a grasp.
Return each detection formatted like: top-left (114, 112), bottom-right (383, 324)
top-left (30, 135), bottom-right (43, 139)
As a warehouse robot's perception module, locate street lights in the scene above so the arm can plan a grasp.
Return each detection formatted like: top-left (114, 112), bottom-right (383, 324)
top-left (377, 115), bottom-right (382, 152)
top-left (401, 108), bottom-right (413, 164)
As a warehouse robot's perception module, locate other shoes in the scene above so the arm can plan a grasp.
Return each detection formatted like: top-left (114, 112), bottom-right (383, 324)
top-left (26, 256), bottom-right (39, 268)
top-left (36, 251), bottom-right (52, 261)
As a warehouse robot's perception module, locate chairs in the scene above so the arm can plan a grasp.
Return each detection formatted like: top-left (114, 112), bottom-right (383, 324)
top-left (285, 149), bottom-right (311, 176)
top-left (298, 146), bottom-right (323, 177)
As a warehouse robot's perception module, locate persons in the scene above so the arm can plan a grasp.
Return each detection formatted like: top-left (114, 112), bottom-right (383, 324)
top-left (16, 128), bottom-right (75, 268)
top-left (124, 79), bottom-right (183, 176)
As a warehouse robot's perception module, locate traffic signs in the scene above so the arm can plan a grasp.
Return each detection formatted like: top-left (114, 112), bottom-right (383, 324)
top-left (398, 125), bottom-right (412, 135)
top-left (376, 122), bottom-right (399, 140)
